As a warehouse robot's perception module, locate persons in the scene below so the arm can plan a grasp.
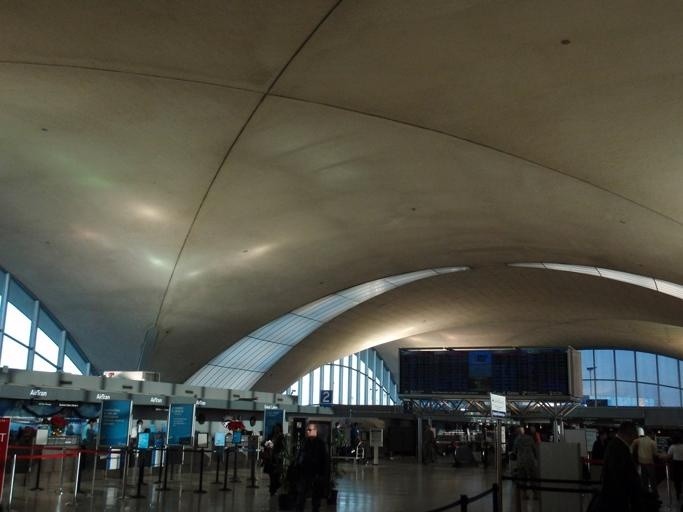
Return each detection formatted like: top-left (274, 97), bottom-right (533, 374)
top-left (423, 415), bottom-right (683, 511)
top-left (350, 422), bottom-right (358, 447)
top-left (244, 415), bottom-right (261, 436)
top-left (129, 418), bottom-right (143, 438)
top-left (332, 421), bottom-right (342, 460)
top-left (297, 420), bottom-right (332, 511)
top-left (259, 422), bottom-right (285, 496)
top-left (80, 418), bottom-right (96, 441)
